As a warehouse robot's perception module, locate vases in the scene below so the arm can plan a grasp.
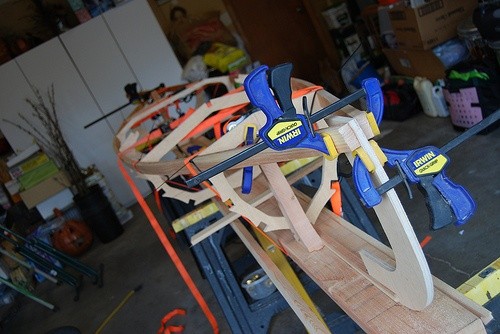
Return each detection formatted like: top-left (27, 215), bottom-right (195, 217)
top-left (72, 184), bottom-right (126, 246)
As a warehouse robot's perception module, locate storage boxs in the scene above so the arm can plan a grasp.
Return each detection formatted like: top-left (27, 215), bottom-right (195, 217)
top-left (0, 142), bottom-right (85, 290)
top-left (376, 0), bottom-right (483, 84)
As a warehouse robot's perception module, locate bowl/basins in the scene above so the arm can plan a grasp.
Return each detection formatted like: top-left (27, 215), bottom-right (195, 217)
top-left (241, 267), bottom-right (277, 300)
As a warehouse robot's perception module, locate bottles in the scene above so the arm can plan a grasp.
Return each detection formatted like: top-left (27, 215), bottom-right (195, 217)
top-left (413, 76), bottom-right (439, 117)
top-left (431, 81), bottom-right (449, 118)
